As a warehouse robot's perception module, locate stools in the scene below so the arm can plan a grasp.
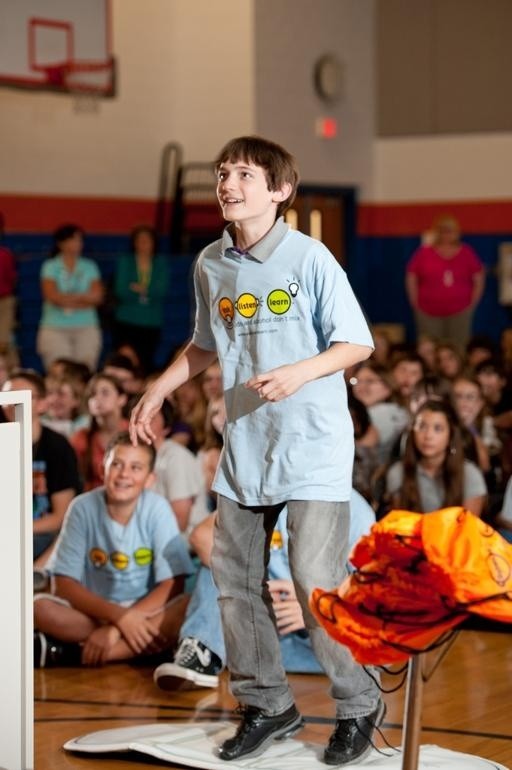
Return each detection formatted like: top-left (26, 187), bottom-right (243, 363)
top-left (403, 608), bottom-right (512, 769)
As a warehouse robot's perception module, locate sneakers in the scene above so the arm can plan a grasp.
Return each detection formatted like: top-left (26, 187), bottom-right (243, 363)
top-left (154, 638), bottom-right (220, 693)
top-left (129, 647), bottom-right (177, 669)
top-left (324, 698), bottom-right (387, 766)
top-left (221, 702), bottom-right (306, 760)
top-left (34, 632), bottom-right (83, 668)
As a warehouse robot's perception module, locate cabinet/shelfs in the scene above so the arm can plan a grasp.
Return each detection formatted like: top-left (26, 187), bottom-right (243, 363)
top-left (1, 389), bottom-right (33, 769)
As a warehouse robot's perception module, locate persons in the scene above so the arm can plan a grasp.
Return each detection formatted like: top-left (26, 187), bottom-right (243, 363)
top-left (405, 218), bottom-right (486, 364)
top-left (128, 136), bottom-right (388, 765)
top-left (36, 222), bottom-right (106, 375)
top-left (492, 241), bottom-right (512, 374)
top-left (0, 212), bottom-right (20, 373)
top-left (114, 224), bottom-right (162, 368)
top-left (0, 320), bottom-right (512, 691)
